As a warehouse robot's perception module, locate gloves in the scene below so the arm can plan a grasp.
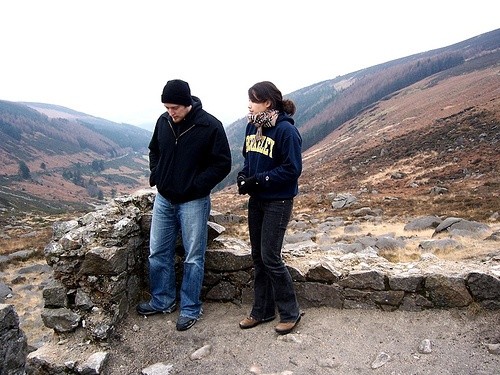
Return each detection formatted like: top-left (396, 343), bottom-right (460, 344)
top-left (237, 176), bottom-right (255, 194)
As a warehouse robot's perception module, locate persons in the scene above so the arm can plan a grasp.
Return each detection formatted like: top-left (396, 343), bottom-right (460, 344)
top-left (136, 79), bottom-right (232, 331)
top-left (237, 81), bottom-right (303, 335)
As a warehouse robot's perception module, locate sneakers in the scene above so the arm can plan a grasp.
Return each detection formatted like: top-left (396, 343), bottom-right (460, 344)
top-left (136, 302), bottom-right (176, 316)
top-left (239, 315), bottom-right (275, 328)
top-left (275, 314), bottom-right (301, 334)
top-left (176, 305), bottom-right (203, 331)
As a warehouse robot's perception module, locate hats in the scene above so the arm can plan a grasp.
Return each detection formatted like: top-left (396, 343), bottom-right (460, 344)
top-left (162, 80), bottom-right (192, 106)
